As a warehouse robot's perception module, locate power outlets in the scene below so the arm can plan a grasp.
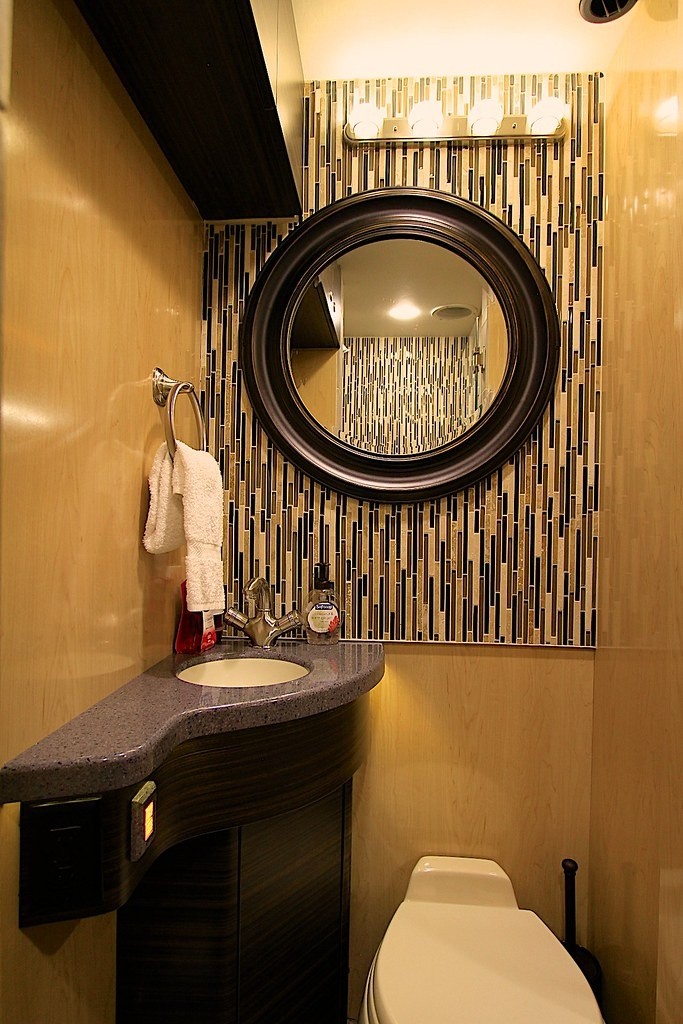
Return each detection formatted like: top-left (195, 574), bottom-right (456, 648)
top-left (131, 780), bottom-right (159, 862)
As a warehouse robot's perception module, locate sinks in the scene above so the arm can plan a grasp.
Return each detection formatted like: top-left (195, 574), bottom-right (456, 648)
top-left (169, 645), bottom-right (315, 690)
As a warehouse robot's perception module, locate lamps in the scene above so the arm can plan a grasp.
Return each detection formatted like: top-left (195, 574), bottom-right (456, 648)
top-left (341, 97), bottom-right (570, 147)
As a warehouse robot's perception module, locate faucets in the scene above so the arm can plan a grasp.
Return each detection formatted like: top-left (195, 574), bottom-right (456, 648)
top-left (241, 576), bottom-right (274, 649)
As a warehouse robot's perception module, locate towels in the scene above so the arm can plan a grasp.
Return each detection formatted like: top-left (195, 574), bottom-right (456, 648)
top-left (141, 438), bottom-right (227, 619)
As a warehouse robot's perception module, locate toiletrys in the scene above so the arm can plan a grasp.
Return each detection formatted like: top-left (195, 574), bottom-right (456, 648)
top-left (302, 562), bottom-right (342, 646)
top-left (174, 554), bottom-right (218, 656)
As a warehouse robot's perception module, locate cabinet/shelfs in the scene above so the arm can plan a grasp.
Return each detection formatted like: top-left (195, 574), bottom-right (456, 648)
top-left (18, 692), bottom-right (371, 1024)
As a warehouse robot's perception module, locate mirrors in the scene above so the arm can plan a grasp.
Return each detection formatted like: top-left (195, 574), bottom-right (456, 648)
top-left (241, 187), bottom-right (560, 502)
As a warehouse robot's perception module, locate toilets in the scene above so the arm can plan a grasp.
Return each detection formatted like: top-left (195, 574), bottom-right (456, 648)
top-left (355, 853), bottom-right (608, 1023)
